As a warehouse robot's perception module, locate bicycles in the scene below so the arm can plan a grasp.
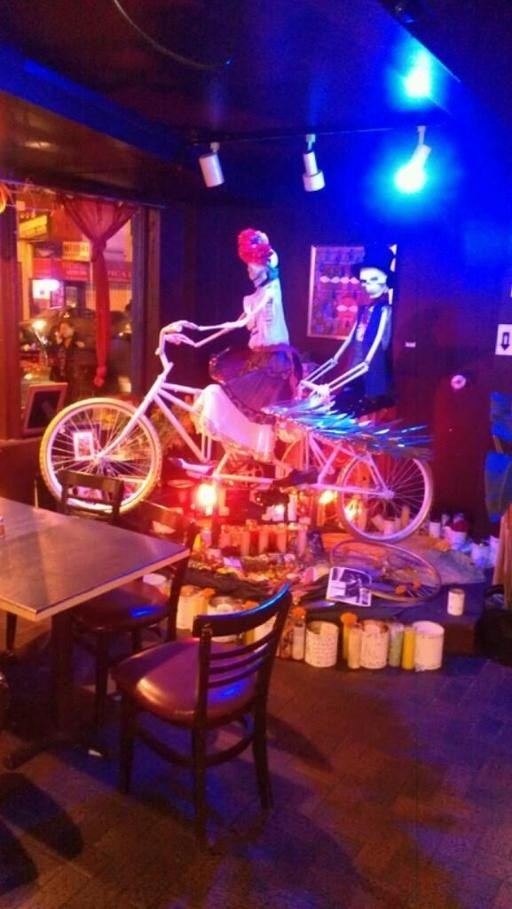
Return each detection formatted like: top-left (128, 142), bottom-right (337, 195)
top-left (39, 325), bottom-right (434, 544)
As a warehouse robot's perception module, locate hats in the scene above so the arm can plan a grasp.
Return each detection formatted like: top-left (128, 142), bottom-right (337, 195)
top-left (239, 229), bottom-right (279, 279)
top-left (352, 239), bottom-right (397, 289)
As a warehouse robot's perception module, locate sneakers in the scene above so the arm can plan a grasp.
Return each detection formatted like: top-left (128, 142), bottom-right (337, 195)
top-left (272, 468), bottom-right (319, 486)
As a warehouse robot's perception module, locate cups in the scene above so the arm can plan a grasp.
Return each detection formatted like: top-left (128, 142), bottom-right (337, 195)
top-left (340, 612), bottom-right (362, 669)
top-left (428, 520), bottom-right (441, 540)
top-left (291, 606), bottom-right (306, 661)
top-left (390, 621), bottom-right (416, 669)
top-left (447, 584), bottom-right (467, 617)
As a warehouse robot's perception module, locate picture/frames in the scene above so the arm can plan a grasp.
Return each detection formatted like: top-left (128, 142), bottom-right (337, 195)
top-left (21, 382), bottom-right (68, 436)
top-left (306, 245), bottom-right (398, 343)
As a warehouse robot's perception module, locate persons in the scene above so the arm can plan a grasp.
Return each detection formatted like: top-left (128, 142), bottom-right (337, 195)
top-left (53, 322), bottom-right (89, 381)
top-left (88, 310), bottom-right (128, 395)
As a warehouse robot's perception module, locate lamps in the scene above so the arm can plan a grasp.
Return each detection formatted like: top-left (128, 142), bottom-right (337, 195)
top-left (193, 123), bottom-right (433, 193)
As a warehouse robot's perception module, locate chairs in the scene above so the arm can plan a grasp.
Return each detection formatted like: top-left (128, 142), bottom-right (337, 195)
top-left (6, 472), bottom-right (125, 652)
top-left (113, 582), bottom-right (292, 841)
top-left (59, 499), bottom-right (202, 755)
